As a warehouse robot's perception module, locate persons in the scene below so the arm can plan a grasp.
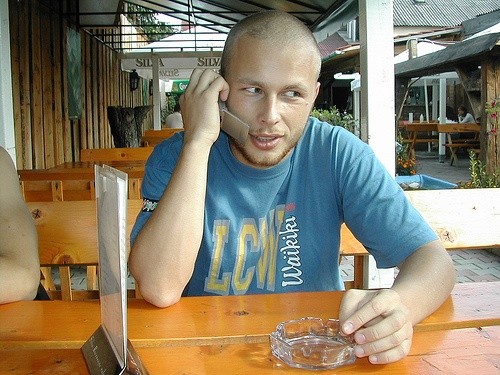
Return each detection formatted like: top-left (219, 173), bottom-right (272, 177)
top-left (456, 104), bottom-right (476, 140)
top-left (165, 103), bottom-right (184, 129)
top-left (128, 9), bottom-right (456, 365)
top-left (0, 144), bottom-right (41, 306)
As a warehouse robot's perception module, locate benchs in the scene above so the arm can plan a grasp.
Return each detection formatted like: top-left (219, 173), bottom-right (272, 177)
top-left (401, 122), bottom-right (439, 160)
top-left (436, 123), bottom-right (481, 168)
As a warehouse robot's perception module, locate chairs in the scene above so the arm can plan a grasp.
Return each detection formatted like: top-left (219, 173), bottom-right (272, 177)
top-left (13, 129), bottom-right (186, 300)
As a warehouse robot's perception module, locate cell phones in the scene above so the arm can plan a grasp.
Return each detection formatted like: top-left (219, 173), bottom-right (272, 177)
top-left (218, 98), bottom-right (250, 144)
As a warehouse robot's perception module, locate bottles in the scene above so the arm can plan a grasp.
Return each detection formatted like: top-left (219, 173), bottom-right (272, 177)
top-left (420, 113), bottom-right (424, 123)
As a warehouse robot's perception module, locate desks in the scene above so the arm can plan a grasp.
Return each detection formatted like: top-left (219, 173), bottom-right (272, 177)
top-left (46, 161), bottom-right (145, 197)
top-left (0, 280), bottom-right (500, 374)
